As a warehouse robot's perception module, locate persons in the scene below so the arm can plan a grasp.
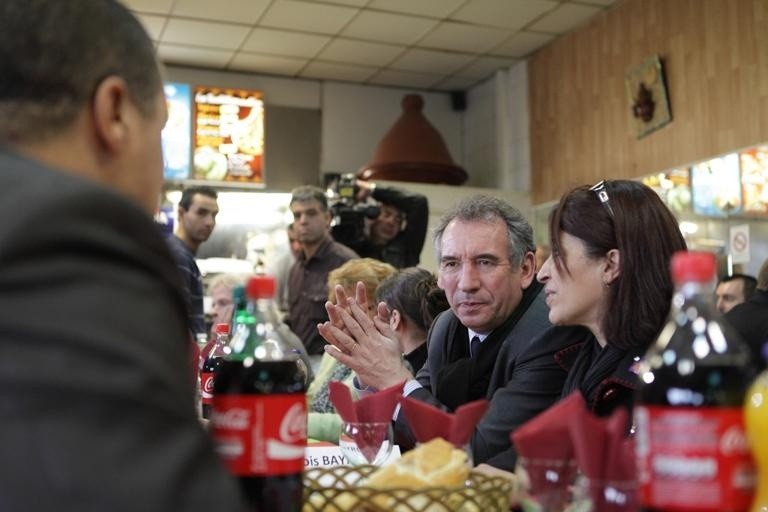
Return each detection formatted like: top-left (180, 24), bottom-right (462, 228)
top-left (168, 184), bottom-right (220, 348)
top-left (719, 257), bottom-right (767, 387)
top-left (371, 264), bottom-right (452, 455)
top-left (312, 193), bottom-right (601, 471)
top-left (0, 0), bottom-right (258, 511)
top-left (272, 220), bottom-right (304, 323)
top-left (287, 184), bottom-right (362, 358)
top-left (302, 254), bottom-right (399, 446)
top-left (710, 265), bottom-right (759, 316)
top-left (325, 161), bottom-right (430, 272)
top-left (482, 176), bottom-right (763, 477)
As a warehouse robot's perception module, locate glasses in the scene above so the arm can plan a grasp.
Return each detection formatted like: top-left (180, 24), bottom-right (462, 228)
top-left (587, 177), bottom-right (615, 221)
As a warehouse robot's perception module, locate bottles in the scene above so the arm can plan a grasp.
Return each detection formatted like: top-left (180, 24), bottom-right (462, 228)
top-left (200, 278), bottom-right (305, 512)
top-left (631, 252), bottom-right (756, 512)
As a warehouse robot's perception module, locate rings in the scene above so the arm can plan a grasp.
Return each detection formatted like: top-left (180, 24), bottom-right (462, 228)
top-left (349, 342), bottom-right (358, 355)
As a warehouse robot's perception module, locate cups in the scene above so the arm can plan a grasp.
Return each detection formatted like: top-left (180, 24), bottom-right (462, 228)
top-left (340, 422), bottom-right (395, 468)
top-left (514, 457), bottom-right (591, 512)
